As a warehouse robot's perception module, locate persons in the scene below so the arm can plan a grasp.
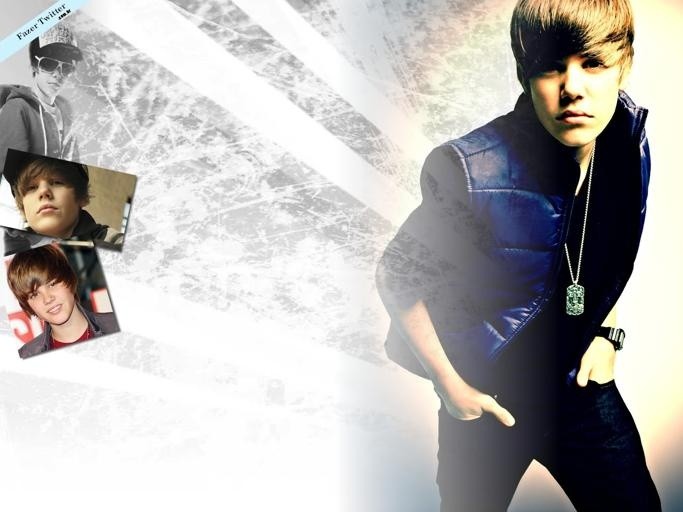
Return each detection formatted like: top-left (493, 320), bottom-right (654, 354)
top-left (3, 151), bottom-right (127, 250)
top-left (363, 1), bottom-right (668, 511)
top-left (4, 234), bottom-right (122, 359)
top-left (0, 19), bottom-right (85, 181)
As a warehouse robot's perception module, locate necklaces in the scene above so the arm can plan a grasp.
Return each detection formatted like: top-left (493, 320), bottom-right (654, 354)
top-left (555, 144), bottom-right (595, 321)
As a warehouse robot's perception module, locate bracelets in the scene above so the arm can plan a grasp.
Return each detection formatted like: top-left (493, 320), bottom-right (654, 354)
top-left (592, 325), bottom-right (627, 349)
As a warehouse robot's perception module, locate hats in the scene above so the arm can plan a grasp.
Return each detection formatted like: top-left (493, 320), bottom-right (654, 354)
top-left (3, 149), bottom-right (89, 197)
top-left (30, 25), bottom-right (82, 62)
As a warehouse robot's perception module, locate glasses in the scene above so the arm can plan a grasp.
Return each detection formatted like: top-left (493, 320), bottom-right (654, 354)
top-left (35, 55), bottom-right (76, 80)
